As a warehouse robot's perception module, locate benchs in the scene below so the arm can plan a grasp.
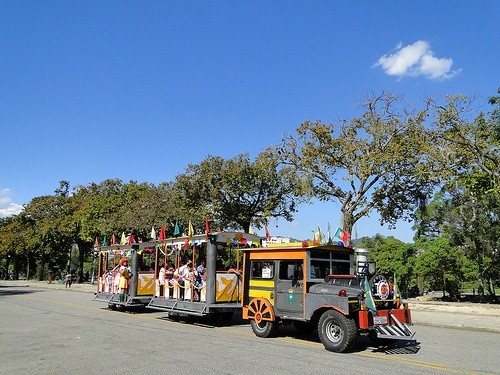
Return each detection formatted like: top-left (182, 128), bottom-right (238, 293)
top-left (99, 277), bottom-right (192, 301)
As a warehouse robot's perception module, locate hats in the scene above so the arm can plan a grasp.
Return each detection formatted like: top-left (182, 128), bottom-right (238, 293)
top-left (120, 258), bottom-right (127, 265)
top-left (187, 260), bottom-right (192, 265)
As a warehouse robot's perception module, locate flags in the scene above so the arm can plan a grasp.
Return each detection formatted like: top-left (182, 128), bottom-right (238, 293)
top-left (364, 276), bottom-right (377, 315)
top-left (128, 233), bottom-right (135, 245)
top-left (110, 234), bottom-right (116, 245)
top-left (392, 274), bottom-right (403, 307)
top-left (335, 228), bottom-right (350, 243)
top-left (95, 236), bottom-right (99, 247)
top-left (174, 219), bottom-right (180, 236)
top-left (205, 217), bottom-right (210, 238)
top-left (160, 225), bottom-right (166, 240)
top-left (103, 234), bottom-right (108, 245)
top-left (264, 226), bottom-right (271, 240)
top-left (249, 226), bottom-right (254, 235)
top-left (121, 232), bottom-right (127, 245)
top-left (188, 220), bottom-right (194, 236)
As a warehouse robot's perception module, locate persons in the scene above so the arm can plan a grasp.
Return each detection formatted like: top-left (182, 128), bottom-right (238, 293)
top-left (64, 271), bottom-right (72, 289)
top-left (119, 258), bottom-right (130, 304)
top-left (159, 256), bottom-right (206, 301)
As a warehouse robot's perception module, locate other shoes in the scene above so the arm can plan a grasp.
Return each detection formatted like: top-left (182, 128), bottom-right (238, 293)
top-left (119, 302), bottom-right (126, 305)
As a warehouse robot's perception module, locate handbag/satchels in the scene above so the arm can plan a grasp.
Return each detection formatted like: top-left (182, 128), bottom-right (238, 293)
top-left (123, 270), bottom-right (129, 278)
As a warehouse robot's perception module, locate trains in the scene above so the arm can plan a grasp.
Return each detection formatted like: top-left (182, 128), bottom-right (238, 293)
top-left (89, 215), bottom-right (421, 353)
